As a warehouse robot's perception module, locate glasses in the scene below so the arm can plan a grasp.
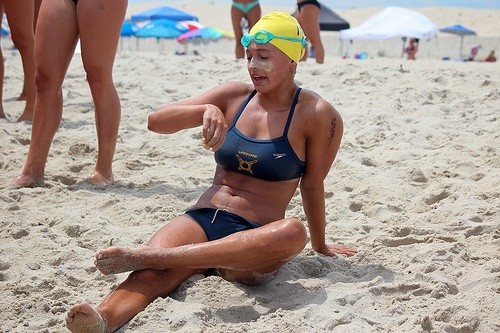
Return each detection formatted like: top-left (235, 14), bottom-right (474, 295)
top-left (240, 30), bottom-right (306, 48)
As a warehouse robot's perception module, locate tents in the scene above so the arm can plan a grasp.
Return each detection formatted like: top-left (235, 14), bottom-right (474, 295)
top-left (117, 6), bottom-right (200, 57)
top-left (318, 4), bottom-right (440, 60)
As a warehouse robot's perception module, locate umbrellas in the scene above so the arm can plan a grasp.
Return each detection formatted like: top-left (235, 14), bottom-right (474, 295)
top-left (440, 25), bottom-right (477, 58)
top-left (176, 21), bottom-right (234, 54)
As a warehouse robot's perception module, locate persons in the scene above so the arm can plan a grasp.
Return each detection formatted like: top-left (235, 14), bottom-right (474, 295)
top-left (64, 11), bottom-right (359, 333)
top-left (293, 0), bottom-right (326, 65)
top-left (0, 0), bottom-right (128, 191)
top-left (404, 40), bottom-right (496, 62)
top-left (229, 0), bottom-right (262, 59)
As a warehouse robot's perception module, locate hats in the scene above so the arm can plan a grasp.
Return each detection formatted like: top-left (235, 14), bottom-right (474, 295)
top-left (249, 12), bottom-right (305, 64)
